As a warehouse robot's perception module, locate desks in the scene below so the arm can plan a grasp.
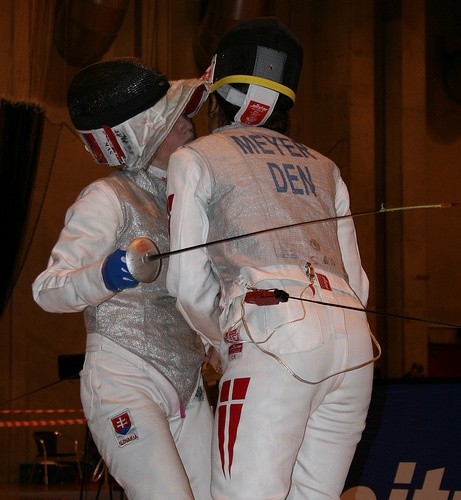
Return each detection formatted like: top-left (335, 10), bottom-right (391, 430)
top-left (340, 377), bottom-right (461, 500)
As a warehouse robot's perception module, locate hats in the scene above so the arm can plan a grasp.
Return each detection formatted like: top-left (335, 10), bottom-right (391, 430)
top-left (67, 58), bottom-right (206, 171)
top-left (215, 18), bottom-right (304, 131)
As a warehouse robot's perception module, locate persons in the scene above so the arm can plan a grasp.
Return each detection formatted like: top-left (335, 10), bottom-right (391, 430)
top-left (32, 57), bottom-right (215, 500)
top-left (167, 18), bottom-right (374, 500)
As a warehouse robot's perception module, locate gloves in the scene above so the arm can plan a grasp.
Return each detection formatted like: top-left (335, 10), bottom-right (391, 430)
top-left (103, 248), bottom-right (138, 291)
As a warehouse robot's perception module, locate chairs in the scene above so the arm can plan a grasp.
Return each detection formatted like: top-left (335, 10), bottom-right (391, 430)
top-left (80, 424), bottom-right (124, 500)
top-left (31, 430), bottom-right (83, 487)
